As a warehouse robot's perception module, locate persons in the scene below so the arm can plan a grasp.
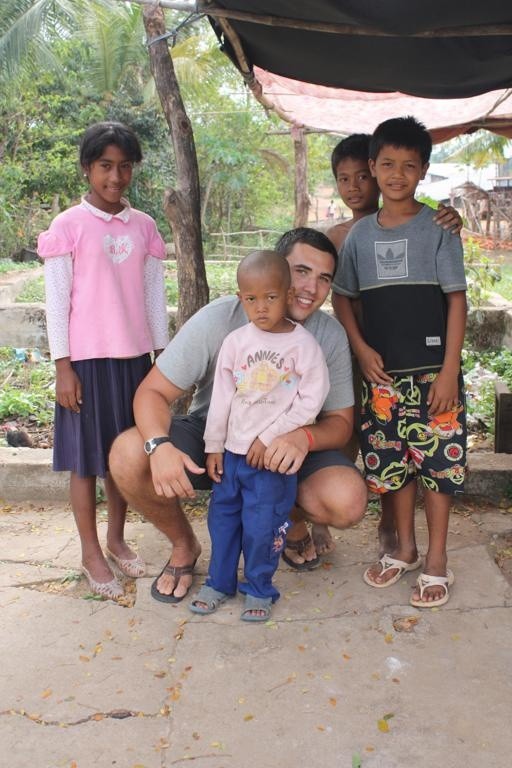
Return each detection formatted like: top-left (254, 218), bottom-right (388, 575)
top-left (326, 200), bottom-right (335, 217)
top-left (332, 116), bottom-right (467, 607)
top-left (311, 134), bottom-right (463, 561)
top-left (107, 226), bottom-right (367, 604)
top-left (37, 121), bottom-right (170, 602)
top-left (476, 197), bottom-right (488, 215)
top-left (187, 250), bottom-right (331, 624)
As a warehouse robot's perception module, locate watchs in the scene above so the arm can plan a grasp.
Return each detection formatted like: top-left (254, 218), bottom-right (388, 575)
top-left (143, 436), bottom-right (173, 456)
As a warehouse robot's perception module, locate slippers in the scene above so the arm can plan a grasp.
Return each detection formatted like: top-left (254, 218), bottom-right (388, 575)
top-left (151, 554), bottom-right (197, 604)
top-left (240, 593), bottom-right (273, 622)
top-left (188, 585), bottom-right (231, 614)
top-left (282, 532), bottom-right (321, 571)
top-left (363, 552), bottom-right (422, 589)
top-left (410, 568), bottom-right (454, 607)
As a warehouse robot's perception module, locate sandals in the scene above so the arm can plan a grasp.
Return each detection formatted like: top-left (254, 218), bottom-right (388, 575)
top-left (80, 559), bottom-right (125, 602)
top-left (105, 545), bottom-right (146, 578)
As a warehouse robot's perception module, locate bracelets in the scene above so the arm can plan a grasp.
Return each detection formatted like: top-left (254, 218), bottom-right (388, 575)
top-left (301, 426), bottom-right (313, 452)
